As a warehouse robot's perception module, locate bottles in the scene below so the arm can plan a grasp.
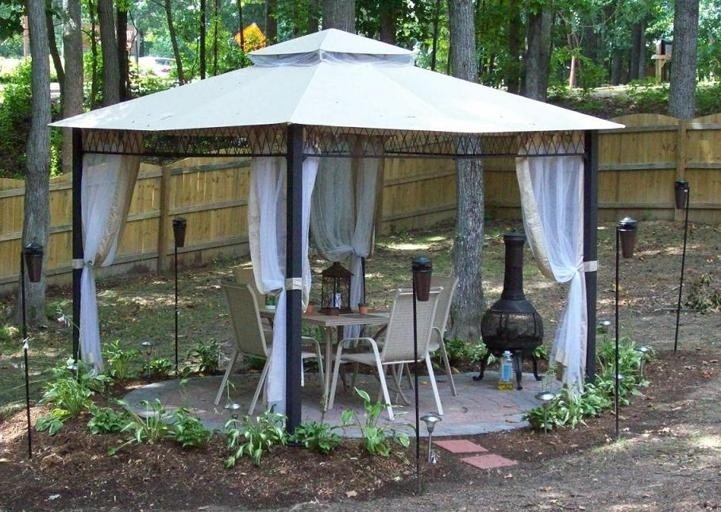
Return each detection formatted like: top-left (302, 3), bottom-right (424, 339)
top-left (498, 350), bottom-right (515, 391)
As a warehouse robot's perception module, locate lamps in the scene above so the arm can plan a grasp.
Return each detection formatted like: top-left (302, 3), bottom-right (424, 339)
top-left (172, 216), bottom-right (187, 378)
top-left (20, 239), bottom-right (44, 458)
top-left (411, 257), bottom-right (432, 479)
top-left (615, 216), bottom-right (639, 431)
top-left (674, 177), bottom-right (690, 350)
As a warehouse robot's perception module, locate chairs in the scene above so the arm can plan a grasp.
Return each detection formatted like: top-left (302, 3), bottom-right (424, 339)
top-left (215, 284), bottom-right (325, 416)
top-left (234, 262), bottom-right (324, 398)
top-left (328, 286), bottom-right (445, 421)
top-left (371, 277), bottom-right (459, 397)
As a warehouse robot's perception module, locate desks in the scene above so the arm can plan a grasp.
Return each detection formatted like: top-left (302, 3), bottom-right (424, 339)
top-left (260, 306), bottom-right (390, 398)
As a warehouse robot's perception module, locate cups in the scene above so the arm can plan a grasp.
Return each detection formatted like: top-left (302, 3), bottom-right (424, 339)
top-left (358, 302), bottom-right (368, 315)
top-left (305, 302), bottom-right (314, 315)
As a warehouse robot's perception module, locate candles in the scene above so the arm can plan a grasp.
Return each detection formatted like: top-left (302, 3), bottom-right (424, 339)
top-left (334, 291), bottom-right (342, 309)
